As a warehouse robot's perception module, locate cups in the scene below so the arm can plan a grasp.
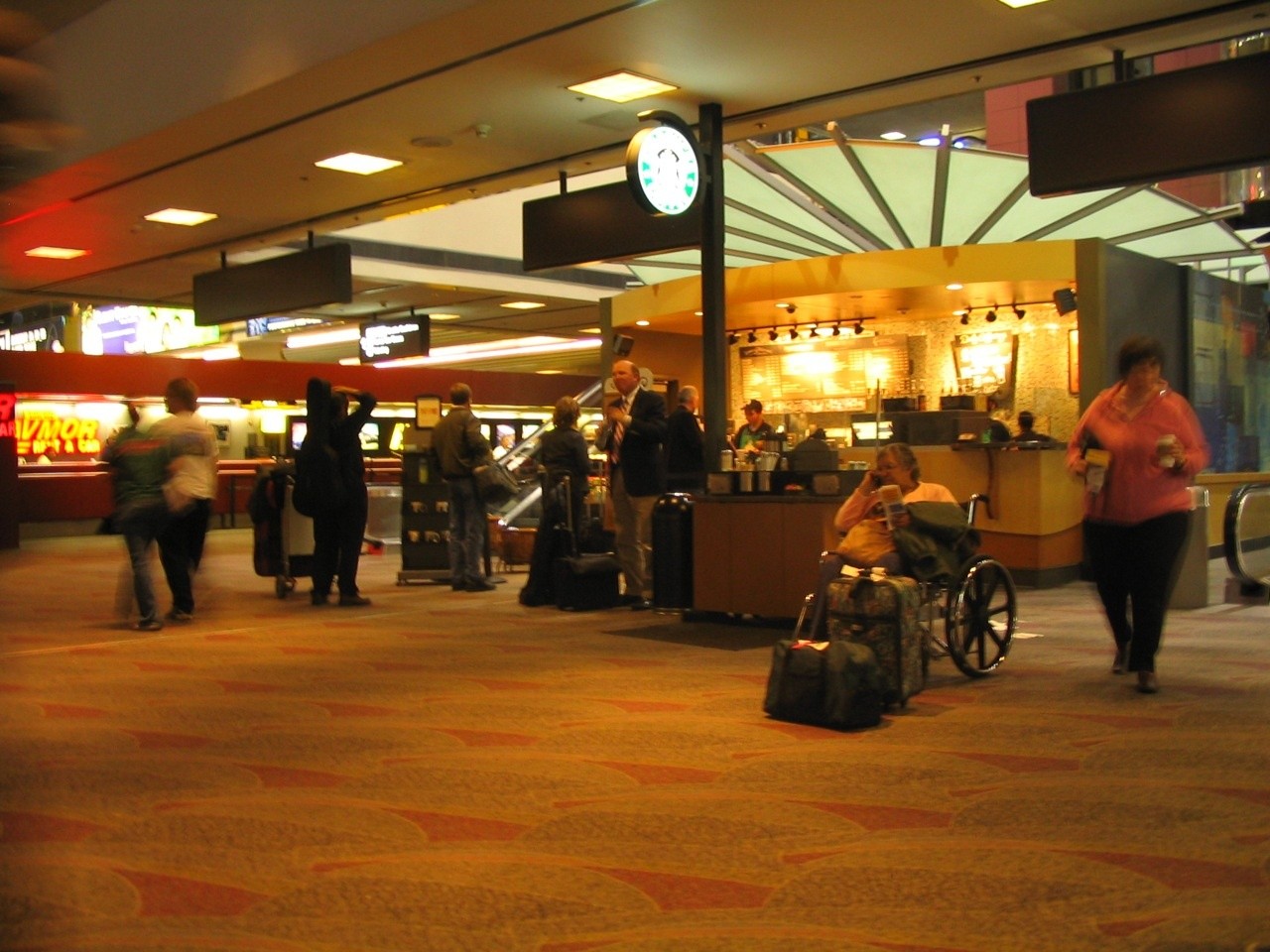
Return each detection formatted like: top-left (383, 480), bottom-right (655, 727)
top-left (758, 472), bottom-right (770, 491)
top-left (1156, 433), bottom-right (1176, 467)
top-left (1085, 448), bottom-right (1111, 493)
top-left (740, 472), bottom-right (752, 491)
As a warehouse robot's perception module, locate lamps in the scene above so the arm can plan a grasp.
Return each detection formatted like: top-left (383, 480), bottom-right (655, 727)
top-left (768, 327), bottom-right (778, 340)
top-left (833, 322), bottom-right (841, 335)
top-left (809, 324), bottom-right (818, 337)
top-left (854, 319), bottom-right (863, 334)
top-left (986, 306), bottom-right (998, 321)
top-left (789, 325), bottom-right (798, 339)
top-left (961, 309), bottom-right (971, 324)
top-left (749, 330), bottom-right (757, 342)
top-left (625, 110), bottom-right (709, 218)
top-left (1013, 306), bottom-right (1025, 319)
top-left (728, 331), bottom-right (739, 345)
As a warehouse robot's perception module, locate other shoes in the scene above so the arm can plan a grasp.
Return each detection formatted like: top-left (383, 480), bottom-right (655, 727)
top-left (632, 598), bottom-right (653, 611)
top-left (134, 618), bottom-right (164, 631)
top-left (618, 594), bottom-right (642, 605)
top-left (1112, 643), bottom-right (1130, 674)
top-left (1138, 671), bottom-right (1160, 693)
top-left (452, 581), bottom-right (497, 591)
top-left (164, 606), bottom-right (193, 620)
top-left (310, 595), bottom-right (329, 605)
top-left (338, 595), bottom-right (372, 607)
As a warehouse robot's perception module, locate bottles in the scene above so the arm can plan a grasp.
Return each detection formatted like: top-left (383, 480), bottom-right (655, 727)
top-left (721, 449), bottom-right (732, 471)
top-left (780, 458), bottom-right (788, 471)
top-left (918, 395), bottom-right (925, 411)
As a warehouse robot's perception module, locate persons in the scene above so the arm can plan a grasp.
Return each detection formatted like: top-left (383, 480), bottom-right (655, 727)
top-left (978, 398), bottom-right (1050, 444)
top-left (97, 376), bottom-right (221, 630)
top-left (426, 381), bottom-right (519, 591)
top-left (1062, 335), bottom-right (1211, 690)
top-left (805, 441), bottom-right (960, 641)
top-left (310, 386), bottom-right (375, 604)
top-left (535, 396), bottom-right (593, 527)
top-left (594, 359), bottom-right (704, 610)
top-left (733, 399), bottom-right (773, 455)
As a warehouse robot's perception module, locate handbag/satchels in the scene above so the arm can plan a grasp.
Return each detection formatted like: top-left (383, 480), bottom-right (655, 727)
top-left (834, 500), bottom-right (897, 568)
top-left (761, 630), bottom-right (884, 732)
top-left (471, 461), bottom-right (523, 502)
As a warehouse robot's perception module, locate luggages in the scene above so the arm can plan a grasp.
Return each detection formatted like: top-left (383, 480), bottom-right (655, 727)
top-left (824, 566), bottom-right (924, 712)
top-left (245, 459), bottom-right (316, 599)
top-left (548, 469), bottom-right (619, 611)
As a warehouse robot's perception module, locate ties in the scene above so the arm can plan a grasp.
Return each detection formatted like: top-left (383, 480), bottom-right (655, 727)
top-left (610, 398), bottom-right (627, 464)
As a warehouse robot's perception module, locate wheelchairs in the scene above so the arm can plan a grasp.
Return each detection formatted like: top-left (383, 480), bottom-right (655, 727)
top-left (790, 492), bottom-right (1019, 679)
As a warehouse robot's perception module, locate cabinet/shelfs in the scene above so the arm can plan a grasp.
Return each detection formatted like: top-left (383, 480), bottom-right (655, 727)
top-left (695, 504), bottom-right (878, 622)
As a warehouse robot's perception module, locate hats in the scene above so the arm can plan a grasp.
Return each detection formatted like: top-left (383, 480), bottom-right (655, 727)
top-left (740, 399), bottom-right (762, 412)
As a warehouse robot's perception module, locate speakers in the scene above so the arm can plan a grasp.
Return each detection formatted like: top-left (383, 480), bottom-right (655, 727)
top-left (615, 334), bottom-right (633, 357)
top-left (1053, 289), bottom-right (1076, 316)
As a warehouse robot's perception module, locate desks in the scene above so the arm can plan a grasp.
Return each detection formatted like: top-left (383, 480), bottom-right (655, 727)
top-left (12, 453), bottom-right (405, 529)
